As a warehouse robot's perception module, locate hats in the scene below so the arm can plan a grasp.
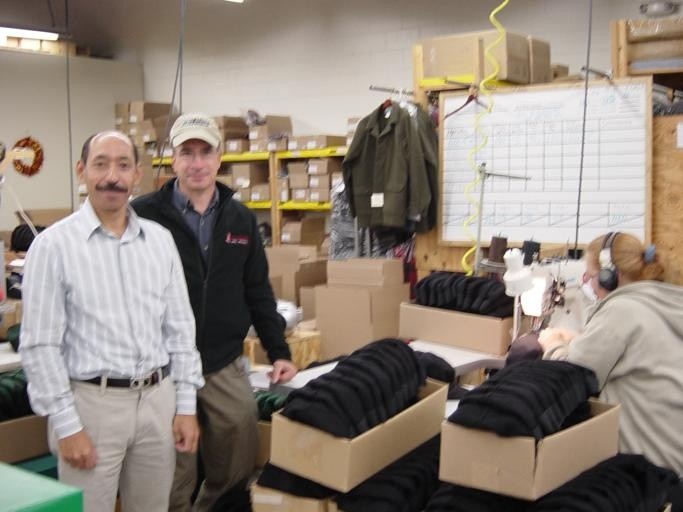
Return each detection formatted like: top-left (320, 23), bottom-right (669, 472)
top-left (169, 111), bottom-right (222, 152)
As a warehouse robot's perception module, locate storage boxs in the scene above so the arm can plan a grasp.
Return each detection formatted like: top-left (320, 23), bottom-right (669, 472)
top-left (109, 98), bottom-right (361, 254)
top-left (0, 229), bottom-right (50, 464)
top-left (412, 28), bottom-right (571, 90)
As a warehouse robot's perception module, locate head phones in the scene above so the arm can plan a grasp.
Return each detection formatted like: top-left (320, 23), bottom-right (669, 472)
top-left (598, 231), bottom-right (618, 292)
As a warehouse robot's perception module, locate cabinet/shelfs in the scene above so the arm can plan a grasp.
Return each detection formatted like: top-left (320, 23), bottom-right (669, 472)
top-left (612, 13), bottom-right (683, 288)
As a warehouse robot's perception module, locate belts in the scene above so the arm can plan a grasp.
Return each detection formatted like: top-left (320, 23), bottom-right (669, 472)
top-left (82, 361), bottom-right (172, 388)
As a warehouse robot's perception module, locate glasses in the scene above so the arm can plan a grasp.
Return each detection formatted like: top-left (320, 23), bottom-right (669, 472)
top-left (581, 272), bottom-right (595, 284)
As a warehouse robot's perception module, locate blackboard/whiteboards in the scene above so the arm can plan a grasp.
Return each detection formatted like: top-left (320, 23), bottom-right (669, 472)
top-left (437, 75), bottom-right (652, 254)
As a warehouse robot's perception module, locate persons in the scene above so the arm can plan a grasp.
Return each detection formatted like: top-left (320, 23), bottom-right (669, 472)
top-left (128, 111), bottom-right (300, 510)
top-left (536, 231), bottom-right (682, 481)
top-left (16, 130), bottom-right (206, 510)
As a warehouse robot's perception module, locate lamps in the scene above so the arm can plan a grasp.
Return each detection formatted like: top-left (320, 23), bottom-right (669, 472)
top-left (0, 1), bottom-right (73, 41)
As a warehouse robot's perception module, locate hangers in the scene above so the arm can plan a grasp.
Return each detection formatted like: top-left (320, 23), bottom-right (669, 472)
top-left (441, 85), bottom-right (495, 120)
top-left (377, 85), bottom-right (417, 120)
